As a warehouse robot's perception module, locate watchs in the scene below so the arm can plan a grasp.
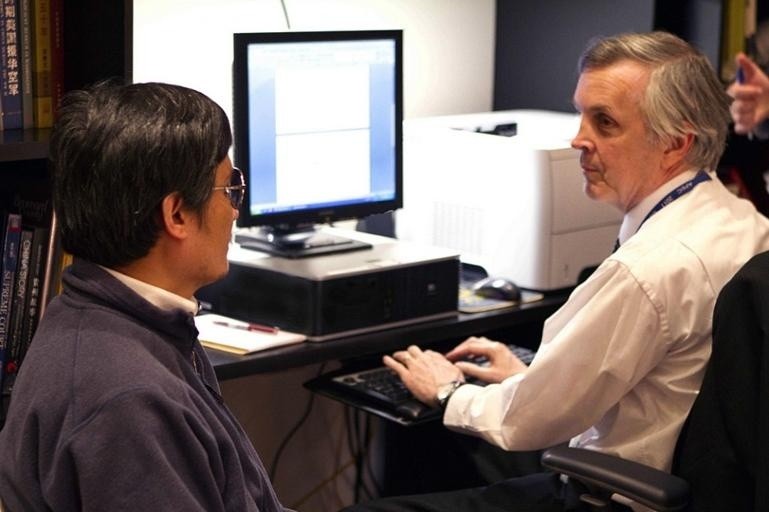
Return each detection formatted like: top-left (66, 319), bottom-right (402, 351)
top-left (432, 380), bottom-right (466, 412)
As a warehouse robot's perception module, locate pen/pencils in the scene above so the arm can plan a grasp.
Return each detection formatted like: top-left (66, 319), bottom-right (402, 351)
top-left (737, 68), bottom-right (753, 142)
top-left (213, 321), bottom-right (274, 333)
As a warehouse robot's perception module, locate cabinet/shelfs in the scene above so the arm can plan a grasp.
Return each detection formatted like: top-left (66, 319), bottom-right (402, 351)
top-left (0, 0), bottom-right (132, 433)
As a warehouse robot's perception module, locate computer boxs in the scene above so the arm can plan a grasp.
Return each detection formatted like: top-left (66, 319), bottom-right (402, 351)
top-left (193, 230), bottom-right (461, 342)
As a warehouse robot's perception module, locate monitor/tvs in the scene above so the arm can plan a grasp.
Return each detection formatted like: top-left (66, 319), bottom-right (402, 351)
top-left (232, 29), bottom-right (402, 262)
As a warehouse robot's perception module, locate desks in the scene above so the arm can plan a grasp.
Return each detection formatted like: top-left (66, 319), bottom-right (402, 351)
top-left (194, 265), bottom-right (574, 381)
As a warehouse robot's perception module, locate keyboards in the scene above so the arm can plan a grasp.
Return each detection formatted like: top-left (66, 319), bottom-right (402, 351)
top-left (331, 344), bottom-right (537, 407)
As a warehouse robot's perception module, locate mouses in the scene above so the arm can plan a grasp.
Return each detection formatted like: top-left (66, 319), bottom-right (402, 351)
top-left (472, 276), bottom-right (521, 301)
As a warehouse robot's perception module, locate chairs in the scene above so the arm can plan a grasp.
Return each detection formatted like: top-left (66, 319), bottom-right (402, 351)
top-left (540, 249), bottom-right (769, 512)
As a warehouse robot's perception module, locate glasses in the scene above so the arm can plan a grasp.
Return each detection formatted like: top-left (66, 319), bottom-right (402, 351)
top-left (210, 166), bottom-right (248, 210)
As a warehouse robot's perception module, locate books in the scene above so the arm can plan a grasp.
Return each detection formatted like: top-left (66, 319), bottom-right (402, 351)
top-left (189, 313), bottom-right (307, 356)
top-left (0, 1), bottom-right (88, 424)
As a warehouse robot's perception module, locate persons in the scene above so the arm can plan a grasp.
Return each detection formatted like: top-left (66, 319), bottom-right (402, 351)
top-left (0, 80), bottom-right (296, 510)
top-left (718, 2), bottom-right (769, 222)
top-left (339, 28), bottom-right (767, 510)
